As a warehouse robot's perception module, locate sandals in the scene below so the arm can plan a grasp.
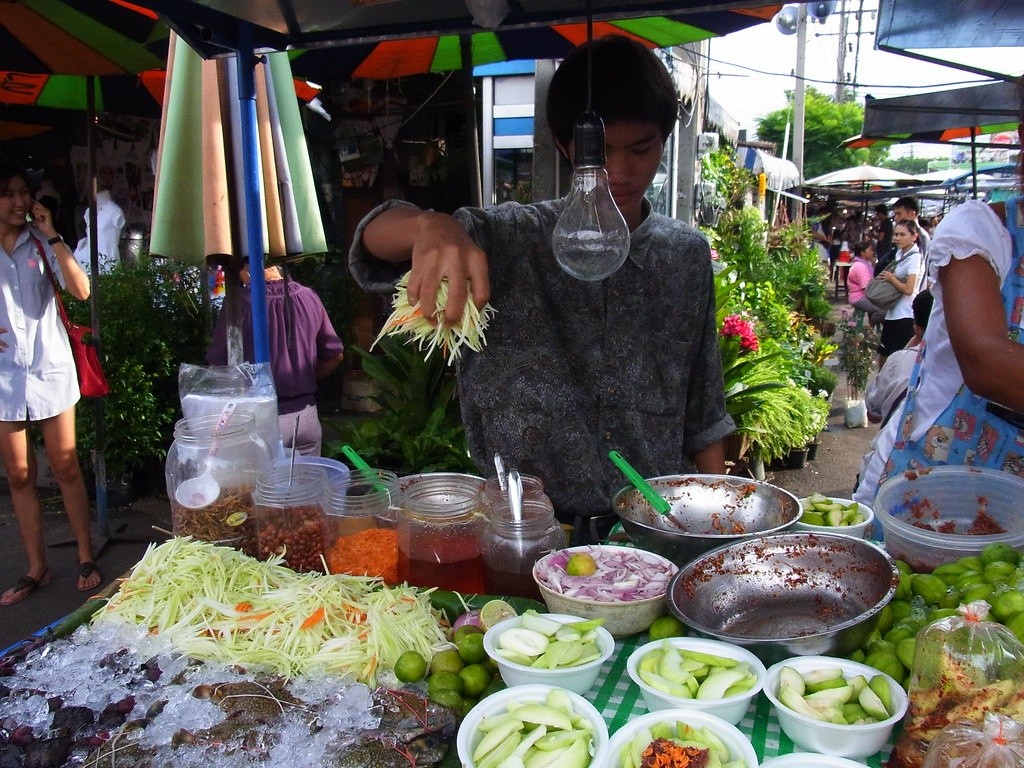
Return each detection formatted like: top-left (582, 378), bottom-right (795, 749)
top-left (77, 560), bottom-right (101, 592)
top-left (1, 568), bottom-right (52, 606)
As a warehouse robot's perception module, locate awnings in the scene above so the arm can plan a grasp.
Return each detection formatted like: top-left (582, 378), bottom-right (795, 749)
top-left (653, 47), bottom-right (740, 149)
top-left (731, 145), bottom-right (801, 190)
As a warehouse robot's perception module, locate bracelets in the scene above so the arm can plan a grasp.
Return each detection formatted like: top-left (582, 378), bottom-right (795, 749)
top-left (47, 234), bottom-right (64, 246)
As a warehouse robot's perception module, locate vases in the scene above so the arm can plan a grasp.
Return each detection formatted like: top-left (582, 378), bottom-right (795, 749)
top-left (728, 441), bottom-right (820, 482)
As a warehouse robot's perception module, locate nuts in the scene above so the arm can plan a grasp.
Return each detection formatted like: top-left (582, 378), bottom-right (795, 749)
top-left (259, 502), bottom-right (326, 574)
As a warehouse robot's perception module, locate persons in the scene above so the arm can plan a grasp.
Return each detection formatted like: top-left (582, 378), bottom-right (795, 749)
top-left (206, 254), bottom-right (345, 458)
top-left (0, 162), bottom-right (101, 606)
top-left (83, 189), bottom-right (125, 275)
top-left (348, 33), bottom-right (737, 549)
top-left (852, 74), bottom-right (1024, 542)
top-left (806, 196), bottom-right (940, 493)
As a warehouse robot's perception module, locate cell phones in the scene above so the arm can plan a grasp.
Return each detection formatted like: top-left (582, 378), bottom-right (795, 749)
top-left (28, 205), bottom-right (36, 220)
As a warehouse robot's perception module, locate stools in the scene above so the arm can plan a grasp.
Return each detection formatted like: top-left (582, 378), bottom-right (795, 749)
top-left (852, 307), bottom-right (882, 335)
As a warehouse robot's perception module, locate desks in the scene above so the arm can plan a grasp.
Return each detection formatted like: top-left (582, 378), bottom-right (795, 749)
top-left (833, 259), bottom-right (853, 302)
top-left (1, 523), bottom-right (900, 768)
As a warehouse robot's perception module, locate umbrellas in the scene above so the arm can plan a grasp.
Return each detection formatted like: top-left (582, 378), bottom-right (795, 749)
top-left (348, 4), bottom-right (784, 209)
top-left (803, 162), bottom-right (923, 214)
top-left (0, 0), bottom-right (329, 539)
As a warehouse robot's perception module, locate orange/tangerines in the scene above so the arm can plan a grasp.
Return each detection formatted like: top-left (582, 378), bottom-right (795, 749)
top-left (648, 616), bottom-right (688, 641)
top-left (566, 552), bottom-right (597, 577)
top-left (395, 599), bottom-right (518, 716)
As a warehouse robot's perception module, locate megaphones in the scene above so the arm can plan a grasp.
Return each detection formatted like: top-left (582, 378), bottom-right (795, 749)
top-left (806, 0), bottom-right (837, 24)
top-left (776, 5), bottom-right (798, 35)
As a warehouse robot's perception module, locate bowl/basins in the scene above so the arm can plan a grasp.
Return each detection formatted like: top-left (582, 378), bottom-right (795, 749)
top-left (874, 465), bottom-right (1024, 571)
top-left (789, 497), bottom-right (873, 540)
top-left (531, 544), bottom-right (681, 638)
top-left (611, 474), bottom-right (803, 569)
top-left (665, 530), bottom-right (899, 658)
top-left (456, 613), bottom-right (910, 768)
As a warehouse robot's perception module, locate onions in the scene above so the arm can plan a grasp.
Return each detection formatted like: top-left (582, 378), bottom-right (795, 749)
top-left (535, 542), bottom-right (676, 602)
top-left (452, 610), bottom-right (482, 633)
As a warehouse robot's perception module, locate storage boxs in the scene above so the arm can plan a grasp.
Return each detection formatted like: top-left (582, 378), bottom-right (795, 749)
top-left (839, 240), bottom-right (850, 263)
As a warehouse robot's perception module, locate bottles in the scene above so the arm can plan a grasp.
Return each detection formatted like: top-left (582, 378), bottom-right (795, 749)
top-left (165, 413), bottom-right (567, 598)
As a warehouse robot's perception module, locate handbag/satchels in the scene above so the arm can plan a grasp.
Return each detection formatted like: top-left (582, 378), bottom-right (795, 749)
top-left (67, 323), bottom-right (109, 398)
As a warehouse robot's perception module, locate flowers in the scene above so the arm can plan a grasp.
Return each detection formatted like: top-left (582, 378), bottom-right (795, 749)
top-left (692, 232), bottom-right (839, 460)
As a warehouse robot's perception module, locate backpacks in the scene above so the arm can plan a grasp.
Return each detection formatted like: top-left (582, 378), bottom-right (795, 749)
top-left (865, 250), bottom-right (922, 310)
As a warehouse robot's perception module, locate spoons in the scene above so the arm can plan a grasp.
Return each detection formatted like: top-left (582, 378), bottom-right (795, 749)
top-left (175, 401), bottom-right (240, 509)
